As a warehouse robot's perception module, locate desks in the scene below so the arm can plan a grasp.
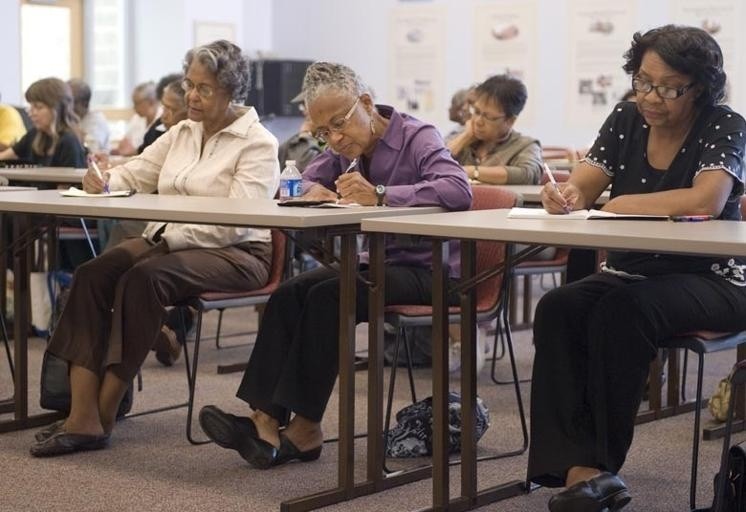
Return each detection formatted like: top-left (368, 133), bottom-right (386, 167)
top-left (361, 207), bottom-right (745, 512)
top-left (1, 186), bottom-right (37, 413)
top-left (476, 185), bottom-right (709, 425)
top-left (1, 167), bottom-right (87, 270)
top-left (0, 190), bottom-right (446, 511)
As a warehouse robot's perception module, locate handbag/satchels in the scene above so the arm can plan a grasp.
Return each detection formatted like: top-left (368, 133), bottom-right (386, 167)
top-left (714, 440), bottom-right (746, 512)
top-left (385, 389), bottom-right (489, 456)
top-left (708, 360), bottom-right (746, 422)
top-left (40, 331), bottom-right (142, 415)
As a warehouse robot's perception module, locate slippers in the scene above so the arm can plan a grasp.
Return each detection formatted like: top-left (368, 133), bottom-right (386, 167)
top-left (35, 419), bottom-right (65, 441)
top-left (200, 405), bottom-right (279, 468)
top-left (30, 416), bottom-right (108, 457)
top-left (275, 431), bottom-right (324, 464)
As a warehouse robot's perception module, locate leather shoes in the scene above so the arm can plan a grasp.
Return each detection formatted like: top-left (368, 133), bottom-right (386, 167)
top-left (548, 471), bottom-right (631, 512)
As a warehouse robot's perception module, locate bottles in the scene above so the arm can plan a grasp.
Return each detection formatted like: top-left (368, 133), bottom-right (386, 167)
top-left (279, 160), bottom-right (302, 200)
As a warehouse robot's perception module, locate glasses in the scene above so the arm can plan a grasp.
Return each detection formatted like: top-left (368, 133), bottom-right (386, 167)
top-left (313, 97), bottom-right (363, 138)
top-left (161, 103), bottom-right (188, 116)
top-left (296, 104), bottom-right (307, 111)
top-left (631, 73), bottom-right (701, 99)
top-left (182, 79), bottom-right (234, 99)
top-left (469, 107), bottom-right (514, 123)
top-left (22, 103), bottom-right (50, 114)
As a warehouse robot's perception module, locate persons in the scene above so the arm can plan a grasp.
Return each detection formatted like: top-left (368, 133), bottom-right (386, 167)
top-left (199, 60), bottom-right (473, 471)
top-left (1, 93), bottom-right (27, 152)
top-left (279, 85), bottom-right (328, 183)
top-left (160, 79), bottom-right (191, 128)
top-left (31, 38), bottom-right (279, 458)
top-left (443, 87), bottom-right (482, 147)
top-left (64, 79), bottom-right (109, 158)
top-left (383, 76), bottom-right (541, 371)
top-left (114, 80), bottom-right (164, 158)
top-left (510, 23), bottom-right (745, 512)
top-left (0, 77), bottom-right (88, 192)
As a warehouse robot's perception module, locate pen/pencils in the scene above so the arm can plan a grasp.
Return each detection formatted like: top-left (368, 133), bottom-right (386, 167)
top-left (336, 158), bottom-right (357, 206)
top-left (544, 162), bottom-right (572, 215)
top-left (669, 213), bottom-right (716, 221)
top-left (88, 157), bottom-right (110, 194)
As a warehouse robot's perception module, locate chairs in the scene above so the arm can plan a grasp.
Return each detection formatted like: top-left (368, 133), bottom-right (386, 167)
top-left (525, 195), bottom-right (746, 512)
top-left (541, 147), bottom-right (573, 174)
top-left (116, 229), bottom-right (287, 446)
top-left (577, 151), bottom-right (588, 159)
top-left (490, 171), bottom-right (571, 385)
top-left (323, 185), bottom-right (528, 473)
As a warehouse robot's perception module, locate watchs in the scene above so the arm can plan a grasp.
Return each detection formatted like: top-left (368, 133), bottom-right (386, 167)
top-left (375, 184), bottom-right (389, 205)
top-left (472, 164), bottom-right (481, 180)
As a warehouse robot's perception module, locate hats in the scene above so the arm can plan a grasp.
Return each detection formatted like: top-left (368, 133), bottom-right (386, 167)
top-left (289, 90), bottom-right (307, 102)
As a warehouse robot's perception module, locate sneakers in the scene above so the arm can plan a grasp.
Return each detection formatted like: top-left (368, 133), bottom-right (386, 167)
top-left (158, 325), bottom-right (182, 365)
